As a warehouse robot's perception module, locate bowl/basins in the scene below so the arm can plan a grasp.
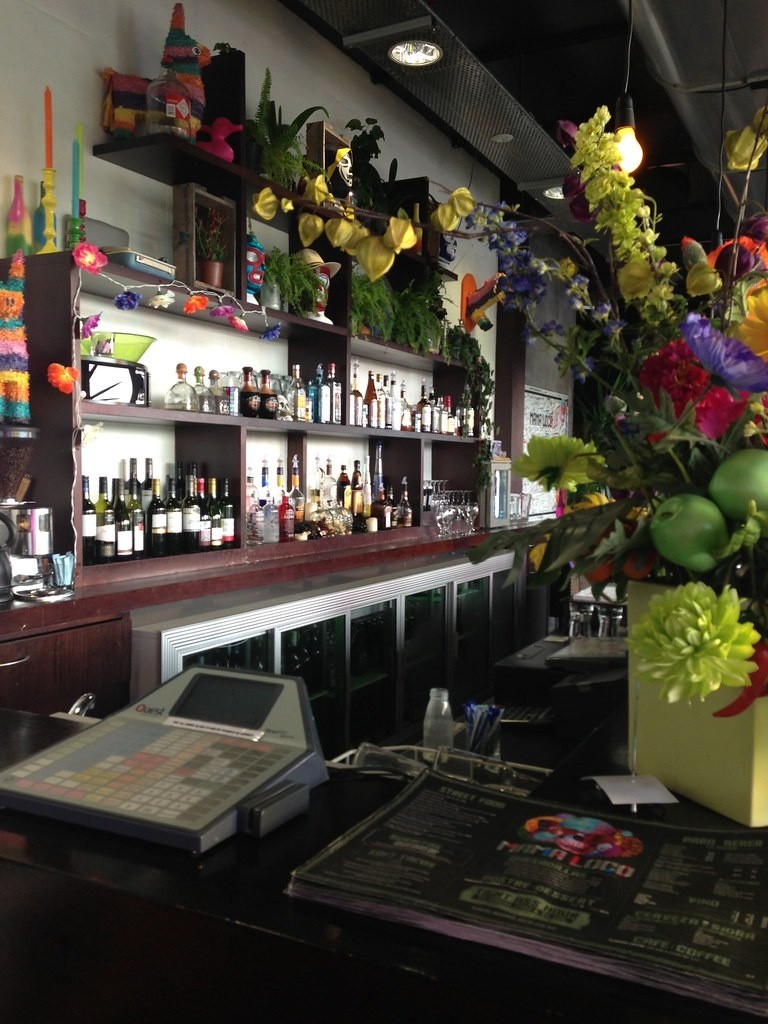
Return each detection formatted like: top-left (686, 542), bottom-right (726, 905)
top-left (82, 326), bottom-right (157, 362)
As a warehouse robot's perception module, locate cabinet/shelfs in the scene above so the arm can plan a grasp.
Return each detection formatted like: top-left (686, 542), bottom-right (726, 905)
top-left (348, 567), bottom-right (449, 747)
top-left (1, 132), bottom-right (491, 588)
top-left (1, 609), bottom-right (132, 726)
top-left (161, 590), bottom-right (348, 761)
top-left (452, 550), bottom-right (527, 714)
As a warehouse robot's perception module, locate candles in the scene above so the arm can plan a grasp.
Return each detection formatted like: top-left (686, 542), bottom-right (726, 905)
top-left (33, 84), bottom-right (91, 218)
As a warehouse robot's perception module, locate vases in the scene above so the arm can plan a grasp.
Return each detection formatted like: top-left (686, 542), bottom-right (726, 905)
top-left (200, 261), bottom-right (224, 289)
top-left (626, 577), bottom-right (768, 828)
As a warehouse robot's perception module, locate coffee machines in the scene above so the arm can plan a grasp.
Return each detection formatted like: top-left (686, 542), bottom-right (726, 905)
top-left (0, 502), bottom-right (74, 608)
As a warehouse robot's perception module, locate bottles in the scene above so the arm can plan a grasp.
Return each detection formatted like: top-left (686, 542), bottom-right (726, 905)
top-left (139, 79), bottom-right (195, 146)
top-left (246, 442), bottom-right (413, 547)
top-left (81, 457), bottom-right (235, 566)
top-left (33, 181), bottom-right (57, 254)
top-left (349, 360), bottom-right (460, 437)
top-left (194, 367), bottom-right (217, 414)
top-left (422, 480), bottom-right (480, 510)
top-left (594, 605), bottom-right (623, 638)
top-left (7, 175), bottom-right (33, 258)
top-left (209, 370), bottom-right (229, 416)
top-left (163, 363), bottom-right (199, 414)
top-left (231, 363), bottom-right (342, 426)
top-left (423, 688), bottom-right (453, 762)
top-left (568, 603), bottom-right (594, 645)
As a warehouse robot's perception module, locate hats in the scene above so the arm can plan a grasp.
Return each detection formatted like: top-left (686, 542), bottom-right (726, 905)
top-left (288, 247), bottom-right (341, 279)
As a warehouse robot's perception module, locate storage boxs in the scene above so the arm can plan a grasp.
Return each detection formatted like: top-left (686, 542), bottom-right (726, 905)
top-left (486, 455), bottom-right (511, 528)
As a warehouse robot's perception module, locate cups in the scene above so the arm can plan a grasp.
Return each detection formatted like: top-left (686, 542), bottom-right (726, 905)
top-left (509, 493), bottom-right (521, 524)
top-left (521, 494), bottom-right (532, 524)
top-left (89, 329), bottom-right (116, 359)
top-left (434, 502), bottom-right (479, 536)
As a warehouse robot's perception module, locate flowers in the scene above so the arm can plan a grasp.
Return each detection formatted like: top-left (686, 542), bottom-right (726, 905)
top-left (193, 203), bottom-right (232, 261)
top-left (249, 98), bottom-right (768, 720)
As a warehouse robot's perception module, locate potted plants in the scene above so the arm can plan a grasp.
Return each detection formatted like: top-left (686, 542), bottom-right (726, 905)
top-left (243, 67), bottom-right (330, 192)
top-left (348, 268), bottom-right (401, 344)
top-left (388, 272), bottom-right (456, 355)
top-left (343, 112), bottom-right (393, 212)
top-left (259, 245), bottom-right (329, 320)
